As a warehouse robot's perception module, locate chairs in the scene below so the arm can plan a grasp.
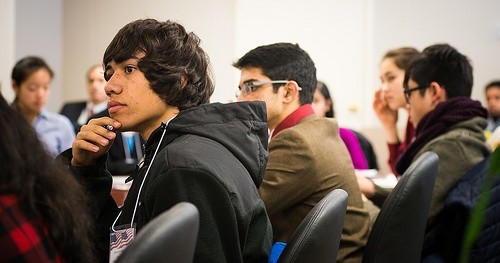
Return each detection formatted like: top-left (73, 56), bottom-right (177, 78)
top-left (363, 151), bottom-right (442, 263)
top-left (274, 188), bottom-right (351, 263)
top-left (113, 202), bottom-right (204, 263)
top-left (422, 150), bottom-right (500, 263)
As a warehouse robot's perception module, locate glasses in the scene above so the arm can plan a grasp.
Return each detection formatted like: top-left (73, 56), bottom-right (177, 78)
top-left (235, 80), bottom-right (302, 101)
top-left (404, 84), bottom-right (430, 104)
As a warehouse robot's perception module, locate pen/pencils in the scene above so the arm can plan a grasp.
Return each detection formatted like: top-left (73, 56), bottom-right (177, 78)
top-left (105, 124), bottom-right (114, 131)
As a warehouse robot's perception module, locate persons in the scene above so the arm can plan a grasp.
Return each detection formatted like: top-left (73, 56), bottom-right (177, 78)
top-left (6, 56), bottom-right (77, 162)
top-left (372, 46), bottom-right (423, 182)
top-left (53, 17), bottom-right (274, 263)
top-left (57, 63), bottom-right (113, 136)
top-left (230, 42), bottom-right (372, 263)
top-left (0, 93), bottom-right (109, 263)
top-left (310, 81), bottom-right (380, 173)
top-left (355, 42), bottom-right (492, 260)
top-left (482, 80), bottom-right (500, 135)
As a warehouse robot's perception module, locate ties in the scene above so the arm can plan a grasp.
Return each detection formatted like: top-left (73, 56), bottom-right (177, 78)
top-left (88, 109), bottom-right (93, 117)
